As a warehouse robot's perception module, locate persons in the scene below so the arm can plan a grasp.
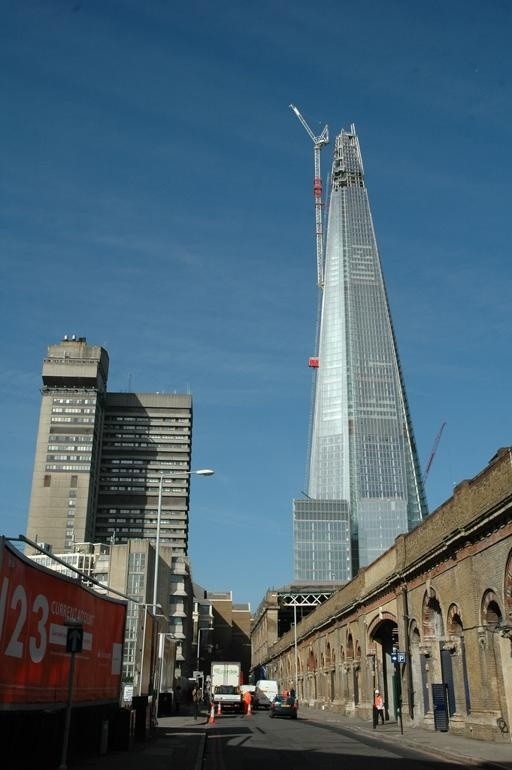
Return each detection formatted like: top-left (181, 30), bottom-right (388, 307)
top-left (242, 688), bottom-right (252, 714)
top-left (191, 686), bottom-right (202, 703)
top-left (174, 685), bottom-right (183, 713)
top-left (374, 689), bottom-right (386, 725)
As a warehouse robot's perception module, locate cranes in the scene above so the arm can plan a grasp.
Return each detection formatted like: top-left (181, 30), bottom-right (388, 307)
top-left (421, 421), bottom-right (447, 485)
top-left (288, 104), bottom-right (329, 287)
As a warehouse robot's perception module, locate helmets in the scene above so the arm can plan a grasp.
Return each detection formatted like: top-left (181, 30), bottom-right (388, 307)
top-left (375, 689), bottom-right (379, 694)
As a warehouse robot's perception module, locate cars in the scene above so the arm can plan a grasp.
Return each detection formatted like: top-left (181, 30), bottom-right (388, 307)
top-left (270, 694), bottom-right (298, 719)
top-left (213, 685), bottom-right (241, 706)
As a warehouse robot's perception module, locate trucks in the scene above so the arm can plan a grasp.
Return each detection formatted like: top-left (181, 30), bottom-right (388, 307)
top-left (211, 661), bottom-right (246, 720)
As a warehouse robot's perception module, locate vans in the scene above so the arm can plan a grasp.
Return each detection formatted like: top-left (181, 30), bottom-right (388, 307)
top-left (253, 679), bottom-right (279, 709)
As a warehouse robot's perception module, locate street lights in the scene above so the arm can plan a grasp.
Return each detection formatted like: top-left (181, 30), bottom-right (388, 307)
top-left (153, 469), bottom-right (214, 615)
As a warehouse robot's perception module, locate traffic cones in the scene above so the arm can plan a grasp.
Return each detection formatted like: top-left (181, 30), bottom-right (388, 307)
top-left (247, 704), bottom-right (251, 715)
top-left (209, 705), bottom-right (215, 723)
top-left (217, 703), bottom-right (221, 714)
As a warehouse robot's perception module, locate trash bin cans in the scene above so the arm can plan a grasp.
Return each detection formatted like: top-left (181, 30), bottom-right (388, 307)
top-left (95, 709), bottom-right (136, 755)
